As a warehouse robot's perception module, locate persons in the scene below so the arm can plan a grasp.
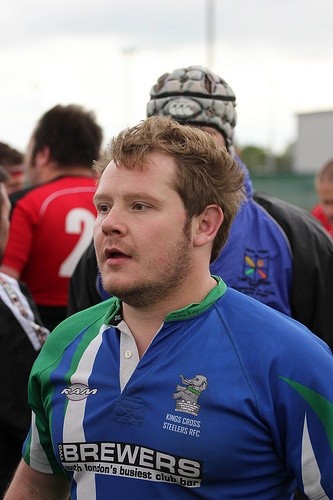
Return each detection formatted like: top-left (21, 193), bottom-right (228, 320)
top-left (148, 66), bottom-right (333, 351)
top-left (2, 114), bottom-right (333, 500)
top-left (0, 104), bottom-right (116, 500)
top-left (312, 158), bottom-right (333, 237)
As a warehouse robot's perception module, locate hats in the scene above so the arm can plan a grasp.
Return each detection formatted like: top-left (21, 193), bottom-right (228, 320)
top-left (146, 66), bottom-right (237, 153)
